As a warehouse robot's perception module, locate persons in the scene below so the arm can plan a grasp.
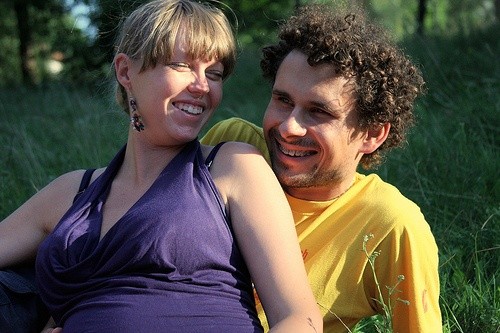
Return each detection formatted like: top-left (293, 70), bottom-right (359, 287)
top-left (0, 0), bottom-right (326, 333)
top-left (43, 0), bottom-right (447, 333)
